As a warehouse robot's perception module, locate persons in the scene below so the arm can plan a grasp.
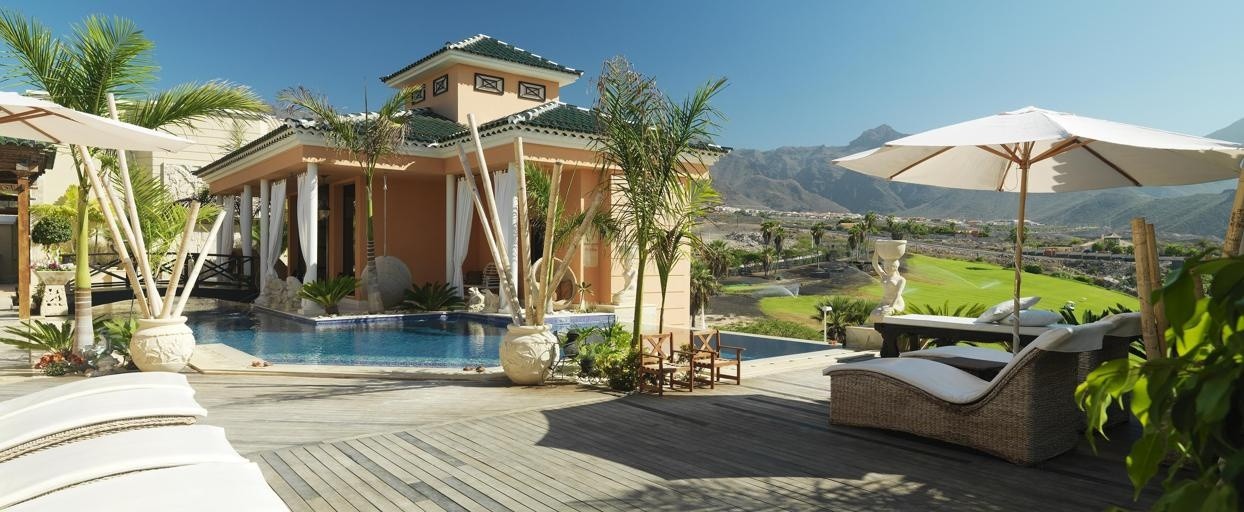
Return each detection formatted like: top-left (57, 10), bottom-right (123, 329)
top-left (864, 238), bottom-right (905, 328)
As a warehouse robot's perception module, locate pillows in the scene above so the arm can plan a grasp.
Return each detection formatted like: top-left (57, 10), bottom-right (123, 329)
top-left (559, 280), bottom-right (572, 300)
top-left (996, 308), bottom-right (1063, 326)
top-left (978, 296), bottom-right (1042, 323)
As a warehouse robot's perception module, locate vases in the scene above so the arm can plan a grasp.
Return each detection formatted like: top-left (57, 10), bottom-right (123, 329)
top-left (129, 316), bottom-right (196, 374)
top-left (498, 322), bottom-right (560, 384)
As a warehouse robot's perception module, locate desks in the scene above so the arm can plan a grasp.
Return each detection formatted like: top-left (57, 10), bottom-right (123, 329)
top-left (872, 310), bottom-right (1050, 370)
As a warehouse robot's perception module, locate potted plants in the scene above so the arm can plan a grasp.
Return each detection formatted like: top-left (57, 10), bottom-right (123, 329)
top-left (31, 212), bottom-right (76, 318)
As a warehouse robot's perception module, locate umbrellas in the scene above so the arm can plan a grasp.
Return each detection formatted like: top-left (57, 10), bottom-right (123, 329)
top-left (0, 91), bottom-right (196, 157)
top-left (828, 106), bottom-right (1244, 358)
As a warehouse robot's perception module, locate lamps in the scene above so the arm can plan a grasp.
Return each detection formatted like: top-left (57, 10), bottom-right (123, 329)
top-left (317, 175), bottom-right (330, 219)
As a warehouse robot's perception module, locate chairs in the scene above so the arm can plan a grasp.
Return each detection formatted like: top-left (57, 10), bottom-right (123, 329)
top-left (823, 313), bottom-right (1142, 467)
top-left (0, 370), bottom-right (286, 512)
top-left (689, 328), bottom-right (747, 389)
top-left (531, 256), bottom-right (579, 311)
top-left (463, 262), bottom-right (501, 301)
top-left (896, 316), bottom-right (1142, 433)
top-left (823, 321), bottom-right (1115, 464)
top-left (640, 331), bottom-right (696, 399)
